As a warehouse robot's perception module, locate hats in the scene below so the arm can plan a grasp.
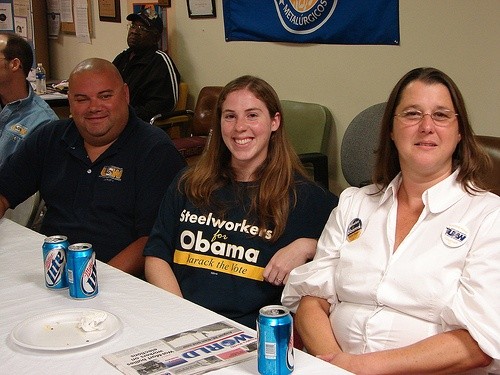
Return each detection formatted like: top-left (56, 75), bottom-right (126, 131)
top-left (126, 8), bottom-right (163, 35)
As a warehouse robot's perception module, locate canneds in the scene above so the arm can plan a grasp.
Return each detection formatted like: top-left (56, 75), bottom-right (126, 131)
top-left (42, 235), bottom-right (70, 291)
top-left (67, 243), bottom-right (98, 299)
top-left (255, 305), bottom-right (295, 375)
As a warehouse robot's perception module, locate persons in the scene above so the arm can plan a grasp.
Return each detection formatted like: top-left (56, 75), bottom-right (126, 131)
top-left (112, 9), bottom-right (180, 121)
top-left (0, 31), bottom-right (59, 164)
top-left (281, 67), bottom-right (500, 375)
top-left (144, 75), bottom-right (338, 330)
top-left (0, 58), bottom-right (180, 276)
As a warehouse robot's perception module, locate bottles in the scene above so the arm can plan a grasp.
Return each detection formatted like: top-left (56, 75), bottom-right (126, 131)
top-left (35, 63), bottom-right (46, 94)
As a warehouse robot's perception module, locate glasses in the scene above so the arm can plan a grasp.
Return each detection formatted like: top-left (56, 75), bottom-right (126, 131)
top-left (393, 109), bottom-right (458, 127)
top-left (127, 23), bottom-right (151, 33)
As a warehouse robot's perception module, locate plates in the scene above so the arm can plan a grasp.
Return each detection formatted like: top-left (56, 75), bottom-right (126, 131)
top-left (10, 307), bottom-right (121, 350)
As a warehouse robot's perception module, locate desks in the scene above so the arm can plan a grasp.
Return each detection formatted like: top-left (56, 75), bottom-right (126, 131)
top-left (33, 78), bottom-right (70, 108)
top-left (0, 216), bottom-right (356, 375)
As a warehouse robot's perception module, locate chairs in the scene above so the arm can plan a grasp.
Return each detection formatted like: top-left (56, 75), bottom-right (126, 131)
top-left (279, 99), bottom-right (332, 191)
top-left (150, 80), bottom-right (225, 170)
top-left (339, 102), bottom-right (500, 198)
